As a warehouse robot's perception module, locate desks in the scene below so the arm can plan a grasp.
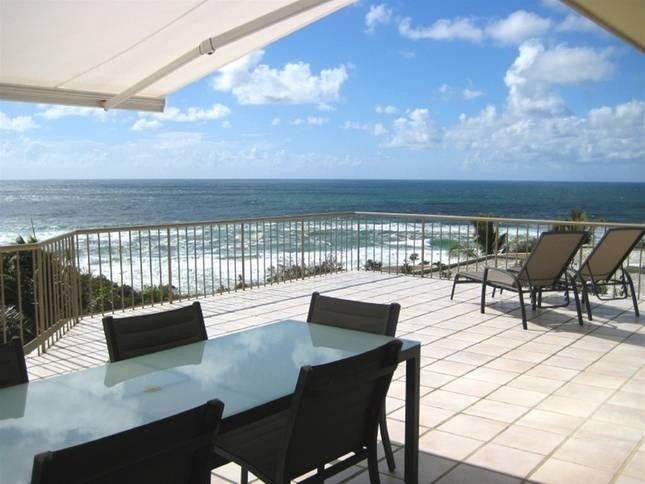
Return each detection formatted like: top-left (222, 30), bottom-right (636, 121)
top-left (0, 318), bottom-right (421, 481)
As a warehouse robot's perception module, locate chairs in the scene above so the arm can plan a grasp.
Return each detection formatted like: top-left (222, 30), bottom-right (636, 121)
top-left (451, 224), bottom-right (645, 330)
